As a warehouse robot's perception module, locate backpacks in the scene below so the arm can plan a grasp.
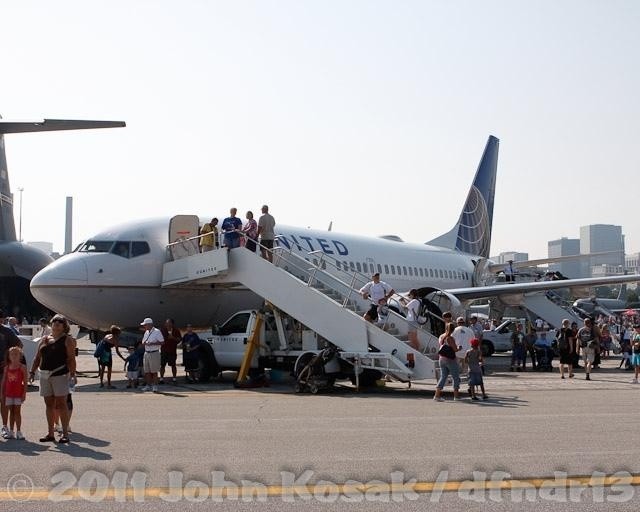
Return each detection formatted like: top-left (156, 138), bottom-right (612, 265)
top-left (559, 330), bottom-right (570, 353)
top-left (412, 298), bottom-right (430, 325)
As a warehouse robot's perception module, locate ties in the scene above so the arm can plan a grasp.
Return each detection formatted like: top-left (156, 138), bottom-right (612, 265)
top-left (510, 264), bottom-right (513, 274)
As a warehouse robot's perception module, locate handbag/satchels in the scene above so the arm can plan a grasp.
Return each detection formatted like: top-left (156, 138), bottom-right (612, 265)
top-left (144, 328), bottom-right (155, 344)
top-left (38, 364), bottom-right (67, 380)
top-left (588, 329), bottom-right (599, 350)
top-left (437, 336), bottom-right (456, 361)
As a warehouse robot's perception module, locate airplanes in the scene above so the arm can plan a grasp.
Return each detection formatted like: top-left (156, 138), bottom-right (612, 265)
top-left (27, 134), bottom-right (640, 392)
top-left (572, 268), bottom-right (640, 319)
top-left (0, 118), bottom-right (127, 320)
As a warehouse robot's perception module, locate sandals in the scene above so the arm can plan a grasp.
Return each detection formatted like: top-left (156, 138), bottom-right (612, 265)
top-left (585, 372), bottom-right (591, 380)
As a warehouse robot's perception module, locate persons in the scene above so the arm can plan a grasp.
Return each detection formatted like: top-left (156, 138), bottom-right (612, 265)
top-left (38, 318), bottom-right (53, 339)
top-left (556, 319), bottom-right (575, 379)
top-left (465, 339), bottom-right (489, 400)
top-left (221, 208), bottom-right (242, 246)
top-left (569, 322), bottom-right (579, 354)
top-left (1, 311), bottom-right (25, 438)
top-left (511, 323), bottom-right (525, 369)
top-left (2, 348), bottom-right (27, 439)
top-left (616, 324), bottom-right (632, 369)
top-left (141, 318), bottom-right (165, 391)
top-left (180, 323), bottom-right (203, 383)
top-left (200, 218), bottom-right (219, 253)
top-left (359, 273), bottom-right (395, 319)
top-left (503, 260), bottom-right (517, 283)
top-left (526, 328), bottom-right (538, 370)
top-left (124, 346), bottom-right (140, 389)
top-left (580, 317), bottom-right (597, 378)
top-left (28, 316), bottom-right (77, 443)
top-left (9, 317), bottom-right (17, 331)
top-left (536, 316), bottom-right (543, 331)
top-left (243, 211), bottom-right (258, 251)
top-left (118, 244), bottom-right (132, 258)
top-left (256, 205), bottom-right (276, 263)
top-left (489, 319), bottom-right (499, 332)
top-left (160, 318), bottom-right (183, 383)
top-left (534, 331), bottom-right (553, 370)
top-left (375, 298), bottom-right (390, 328)
top-left (95, 325), bottom-right (126, 389)
top-left (630, 325), bottom-right (640, 382)
top-left (438, 311), bottom-right (455, 343)
top-left (434, 322), bottom-right (465, 402)
top-left (399, 289), bottom-right (423, 351)
top-left (451, 317), bottom-right (476, 366)
top-left (600, 324), bottom-right (609, 358)
top-left (468, 316), bottom-right (482, 339)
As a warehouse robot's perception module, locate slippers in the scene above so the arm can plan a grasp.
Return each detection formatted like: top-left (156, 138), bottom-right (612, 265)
top-left (99, 384), bottom-right (104, 389)
top-left (107, 385), bottom-right (117, 389)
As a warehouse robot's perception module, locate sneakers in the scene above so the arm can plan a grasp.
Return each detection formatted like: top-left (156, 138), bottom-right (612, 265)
top-left (561, 376), bottom-right (565, 379)
top-left (172, 377), bottom-right (177, 382)
top-left (483, 396), bottom-right (488, 399)
top-left (159, 377), bottom-right (165, 383)
top-left (48, 425), bottom-right (60, 432)
top-left (628, 378), bottom-right (638, 384)
top-left (454, 396), bottom-right (464, 401)
top-left (1, 427), bottom-right (10, 439)
top-left (134, 385), bottom-right (139, 389)
top-left (17, 432), bottom-right (25, 440)
top-left (194, 376), bottom-right (199, 381)
top-left (472, 396), bottom-right (479, 400)
top-left (509, 367), bottom-right (515, 372)
top-left (433, 395), bottom-right (446, 402)
top-left (569, 374), bottom-right (575, 378)
top-left (39, 435), bottom-right (55, 442)
top-left (594, 366), bottom-right (600, 369)
top-left (58, 426), bottom-right (72, 432)
top-left (152, 385), bottom-right (158, 392)
top-left (8, 431), bottom-right (16, 439)
top-left (140, 386), bottom-right (151, 391)
top-left (126, 385), bottom-right (132, 389)
top-left (576, 365), bottom-right (581, 368)
top-left (59, 436), bottom-right (70, 443)
top-left (188, 376), bottom-right (194, 381)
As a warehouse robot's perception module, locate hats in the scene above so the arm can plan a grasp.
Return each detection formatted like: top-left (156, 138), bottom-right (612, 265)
top-left (470, 338), bottom-right (479, 346)
top-left (456, 316), bottom-right (464, 323)
top-left (506, 259), bottom-right (516, 262)
top-left (633, 321), bottom-right (640, 328)
top-left (470, 317), bottom-right (478, 321)
top-left (372, 272), bottom-right (380, 277)
top-left (128, 345), bottom-right (135, 351)
top-left (140, 317), bottom-right (153, 326)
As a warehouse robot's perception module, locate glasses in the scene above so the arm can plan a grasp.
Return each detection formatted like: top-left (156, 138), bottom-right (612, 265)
top-left (143, 325), bottom-right (148, 328)
top-left (509, 262), bottom-right (512, 263)
top-left (186, 326), bottom-right (193, 328)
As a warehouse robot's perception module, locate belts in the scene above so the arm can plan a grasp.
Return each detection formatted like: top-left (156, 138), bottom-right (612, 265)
top-left (144, 350), bottom-right (159, 353)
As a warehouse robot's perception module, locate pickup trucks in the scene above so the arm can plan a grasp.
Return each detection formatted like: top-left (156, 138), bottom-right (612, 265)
top-left (196, 310), bottom-right (332, 375)
top-left (483, 317), bottom-right (556, 356)
